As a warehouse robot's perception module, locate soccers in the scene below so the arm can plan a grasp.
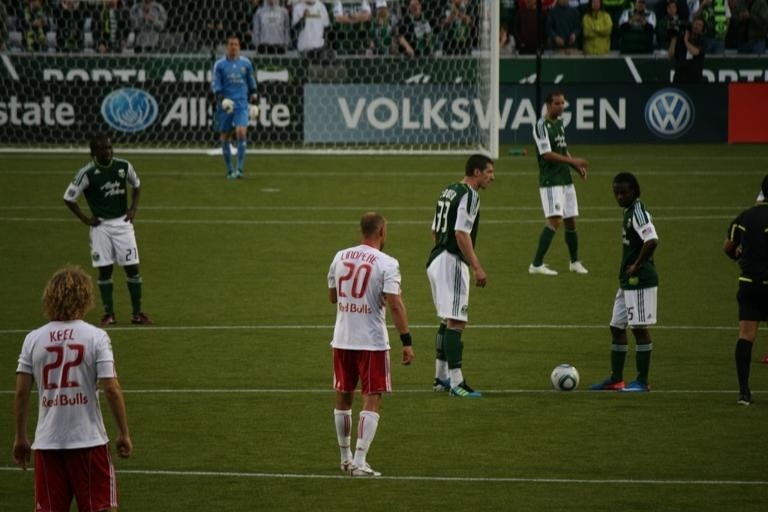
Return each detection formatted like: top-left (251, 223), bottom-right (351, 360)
top-left (551, 364), bottom-right (581, 392)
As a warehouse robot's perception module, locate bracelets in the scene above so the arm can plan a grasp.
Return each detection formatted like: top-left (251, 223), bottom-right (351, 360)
top-left (400, 332), bottom-right (412, 346)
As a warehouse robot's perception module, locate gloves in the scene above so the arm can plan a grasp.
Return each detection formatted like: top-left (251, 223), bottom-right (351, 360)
top-left (222, 98), bottom-right (234, 112)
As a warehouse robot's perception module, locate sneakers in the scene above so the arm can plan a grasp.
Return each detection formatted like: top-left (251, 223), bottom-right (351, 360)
top-left (227, 172), bottom-right (236, 179)
top-left (131, 313), bottom-right (152, 325)
top-left (235, 169), bottom-right (242, 178)
top-left (449, 380), bottom-right (482, 398)
top-left (621, 380), bottom-right (650, 392)
top-left (102, 313), bottom-right (116, 325)
top-left (350, 462), bottom-right (382, 477)
top-left (569, 260), bottom-right (589, 274)
top-left (340, 459), bottom-right (353, 472)
top-left (432, 377), bottom-right (450, 393)
top-left (528, 263), bottom-right (558, 276)
top-left (737, 395), bottom-right (752, 406)
top-left (589, 378), bottom-right (625, 390)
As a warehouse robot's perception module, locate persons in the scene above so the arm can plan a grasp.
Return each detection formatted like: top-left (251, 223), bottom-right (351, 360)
top-left (425, 154), bottom-right (495, 397)
top-left (723, 173), bottom-right (768, 406)
top-left (326, 213), bottom-right (414, 478)
top-left (499, 1), bottom-right (767, 65)
top-left (212, 37), bottom-right (258, 180)
top-left (11, 265), bottom-right (133, 512)
top-left (588, 173), bottom-right (658, 393)
top-left (63, 134), bottom-right (153, 325)
top-left (251, 1), bottom-right (479, 57)
top-left (2, 1), bottom-right (167, 53)
top-left (528, 93), bottom-right (589, 276)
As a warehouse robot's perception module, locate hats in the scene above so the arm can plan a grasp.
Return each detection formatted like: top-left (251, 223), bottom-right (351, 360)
top-left (375, 0), bottom-right (387, 8)
